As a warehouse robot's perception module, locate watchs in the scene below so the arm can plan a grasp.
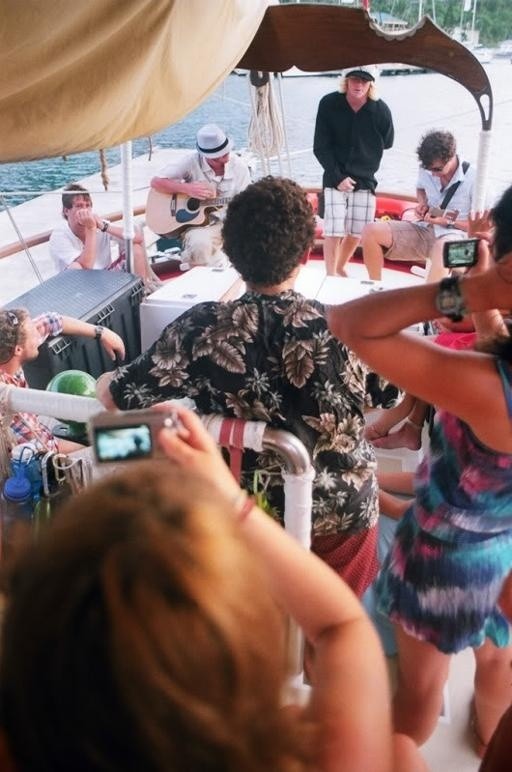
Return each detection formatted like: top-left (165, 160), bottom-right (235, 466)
top-left (100, 219), bottom-right (110, 232)
top-left (435, 273), bottom-right (467, 322)
top-left (94, 326), bottom-right (104, 340)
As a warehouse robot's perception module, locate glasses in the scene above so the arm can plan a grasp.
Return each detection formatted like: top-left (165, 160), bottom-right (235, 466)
top-left (425, 159), bottom-right (448, 171)
top-left (7, 312), bottom-right (18, 347)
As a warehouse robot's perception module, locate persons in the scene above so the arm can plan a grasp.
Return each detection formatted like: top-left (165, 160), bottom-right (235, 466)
top-left (356, 131), bottom-right (512, 525)
top-left (151, 123), bottom-right (253, 224)
top-left (0, 401), bottom-right (395, 772)
top-left (390, 706), bottom-right (512, 772)
top-left (313, 64), bottom-right (393, 278)
top-left (97, 176), bottom-right (404, 600)
top-left (324, 184), bottom-right (512, 759)
top-left (0, 306), bottom-right (126, 457)
top-left (48, 182), bottom-right (152, 286)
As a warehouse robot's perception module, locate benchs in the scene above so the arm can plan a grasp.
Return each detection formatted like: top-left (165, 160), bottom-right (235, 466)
top-left (37, 185), bottom-right (470, 295)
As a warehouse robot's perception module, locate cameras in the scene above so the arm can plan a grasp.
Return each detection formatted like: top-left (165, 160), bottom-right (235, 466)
top-left (88, 411), bottom-right (180, 472)
top-left (443, 238), bottom-right (483, 269)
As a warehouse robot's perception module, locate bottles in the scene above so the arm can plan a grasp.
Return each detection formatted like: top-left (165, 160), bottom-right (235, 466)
top-left (2, 476), bottom-right (32, 523)
top-left (31, 496), bottom-right (51, 537)
top-left (10, 443), bottom-right (42, 501)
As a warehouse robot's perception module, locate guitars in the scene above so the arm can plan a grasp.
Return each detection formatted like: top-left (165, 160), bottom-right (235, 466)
top-left (145, 179), bottom-right (234, 240)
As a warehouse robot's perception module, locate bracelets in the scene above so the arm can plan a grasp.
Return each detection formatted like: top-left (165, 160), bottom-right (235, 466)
top-left (227, 484), bottom-right (255, 527)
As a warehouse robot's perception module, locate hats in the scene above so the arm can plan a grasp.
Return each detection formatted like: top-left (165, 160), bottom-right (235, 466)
top-left (196, 124), bottom-right (233, 158)
top-left (342, 64), bottom-right (378, 82)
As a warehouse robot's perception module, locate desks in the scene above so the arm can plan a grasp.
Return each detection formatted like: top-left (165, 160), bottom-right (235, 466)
top-left (140, 265), bottom-right (244, 353)
